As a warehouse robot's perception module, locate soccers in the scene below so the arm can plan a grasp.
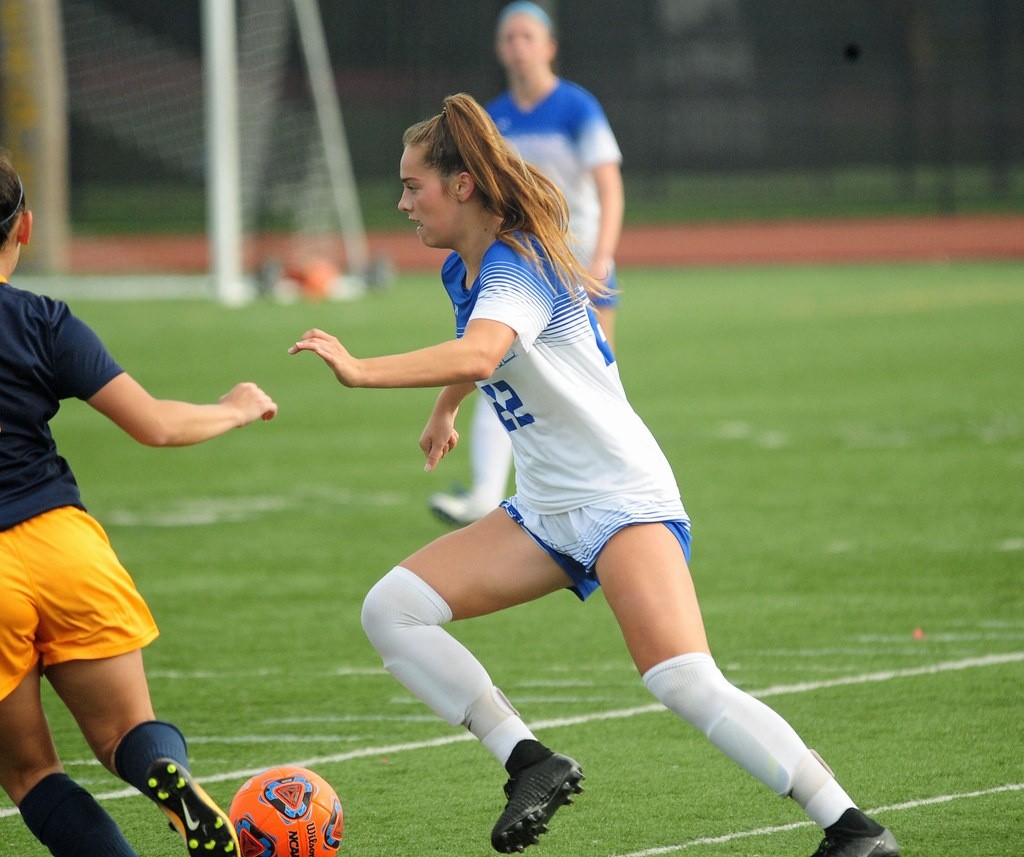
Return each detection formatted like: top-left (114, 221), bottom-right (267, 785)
top-left (227, 766), bottom-right (346, 857)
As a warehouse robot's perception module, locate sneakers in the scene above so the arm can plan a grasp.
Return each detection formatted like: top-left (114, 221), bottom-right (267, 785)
top-left (144, 757), bottom-right (242, 857)
top-left (490, 753), bottom-right (586, 855)
top-left (810, 828), bottom-right (903, 857)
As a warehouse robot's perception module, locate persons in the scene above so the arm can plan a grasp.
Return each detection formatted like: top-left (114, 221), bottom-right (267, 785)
top-left (430, 0), bottom-right (626, 528)
top-left (288, 93), bottom-right (906, 857)
top-left (0, 159), bottom-right (278, 857)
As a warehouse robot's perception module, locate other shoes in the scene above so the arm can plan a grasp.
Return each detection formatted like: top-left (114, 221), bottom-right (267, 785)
top-left (429, 488), bottom-right (503, 528)
